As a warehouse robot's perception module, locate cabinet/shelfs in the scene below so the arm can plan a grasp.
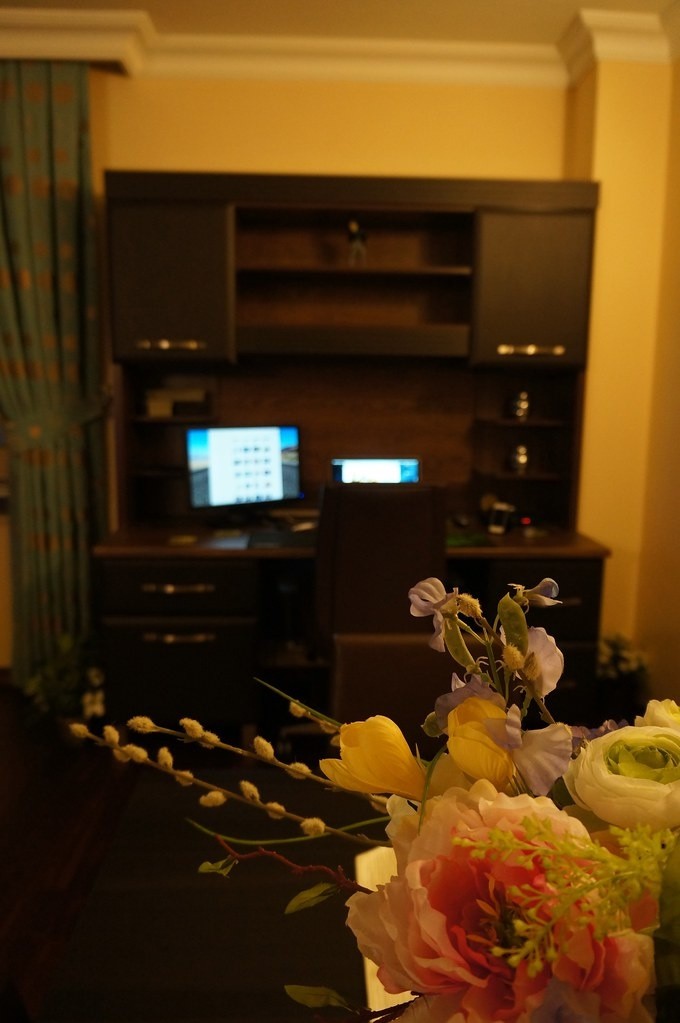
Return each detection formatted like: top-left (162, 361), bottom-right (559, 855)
top-left (102, 167), bottom-right (601, 537)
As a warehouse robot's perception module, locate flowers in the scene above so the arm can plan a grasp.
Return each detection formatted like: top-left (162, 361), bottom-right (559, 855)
top-left (61, 572), bottom-right (680, 1023)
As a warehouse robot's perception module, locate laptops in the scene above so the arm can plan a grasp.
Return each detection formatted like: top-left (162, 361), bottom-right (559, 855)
top-left (329, 451), bottom-right (424, 484)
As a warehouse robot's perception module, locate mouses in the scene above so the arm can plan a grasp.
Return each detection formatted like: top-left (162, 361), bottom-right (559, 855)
top-left (453, 516), bottom-right (469, 527)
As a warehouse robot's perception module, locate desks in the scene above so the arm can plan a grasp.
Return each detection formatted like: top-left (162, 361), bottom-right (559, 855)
top-left (94, 529), bottom-right (613, 745)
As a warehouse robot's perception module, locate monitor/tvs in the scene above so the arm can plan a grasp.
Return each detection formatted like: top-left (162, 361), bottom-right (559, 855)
top-left (181, 423), bottom-right (304, 531)
